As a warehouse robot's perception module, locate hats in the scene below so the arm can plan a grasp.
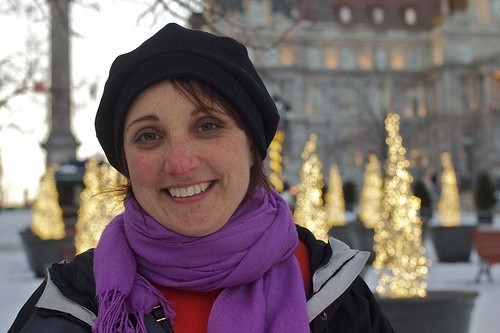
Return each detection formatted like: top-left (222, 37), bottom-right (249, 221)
top-left (94, 22), bottom-right (280, 179)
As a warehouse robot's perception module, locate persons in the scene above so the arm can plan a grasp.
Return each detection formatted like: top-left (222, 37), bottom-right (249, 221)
top-left (8, 24), bottom-right (400, 333)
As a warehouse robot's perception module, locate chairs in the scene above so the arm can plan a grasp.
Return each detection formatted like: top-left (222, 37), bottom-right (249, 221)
top-left (471, 226), bottom-right (500, 285)
